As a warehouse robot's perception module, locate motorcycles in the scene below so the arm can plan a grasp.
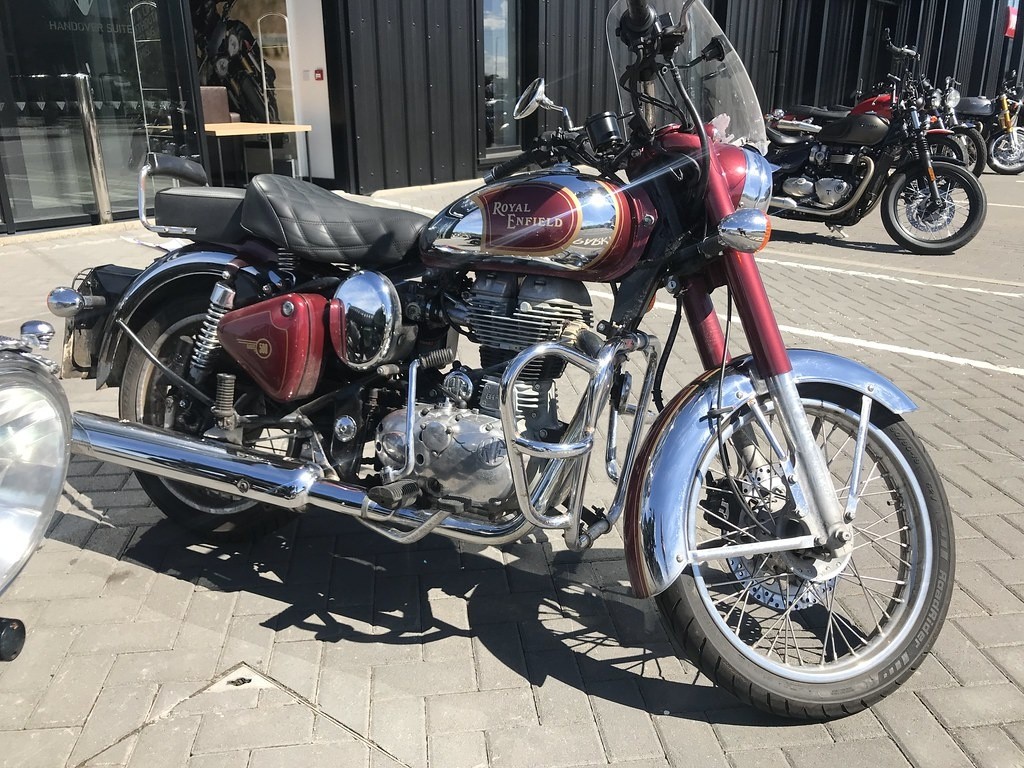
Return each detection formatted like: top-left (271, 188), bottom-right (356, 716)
top-left (728, 25), bottom-right (988, 260)
top-left (41, 1), bottom-right (960, 723)
top-left (795, 67), bottom-right (1024, 186)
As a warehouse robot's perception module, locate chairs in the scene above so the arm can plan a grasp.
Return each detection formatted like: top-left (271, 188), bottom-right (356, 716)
top-left (199, 85), bottom-right (247, 189)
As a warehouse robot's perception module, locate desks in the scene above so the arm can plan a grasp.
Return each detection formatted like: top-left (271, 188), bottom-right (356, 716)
top-left (147, 122), bottom-right (313, 188)
